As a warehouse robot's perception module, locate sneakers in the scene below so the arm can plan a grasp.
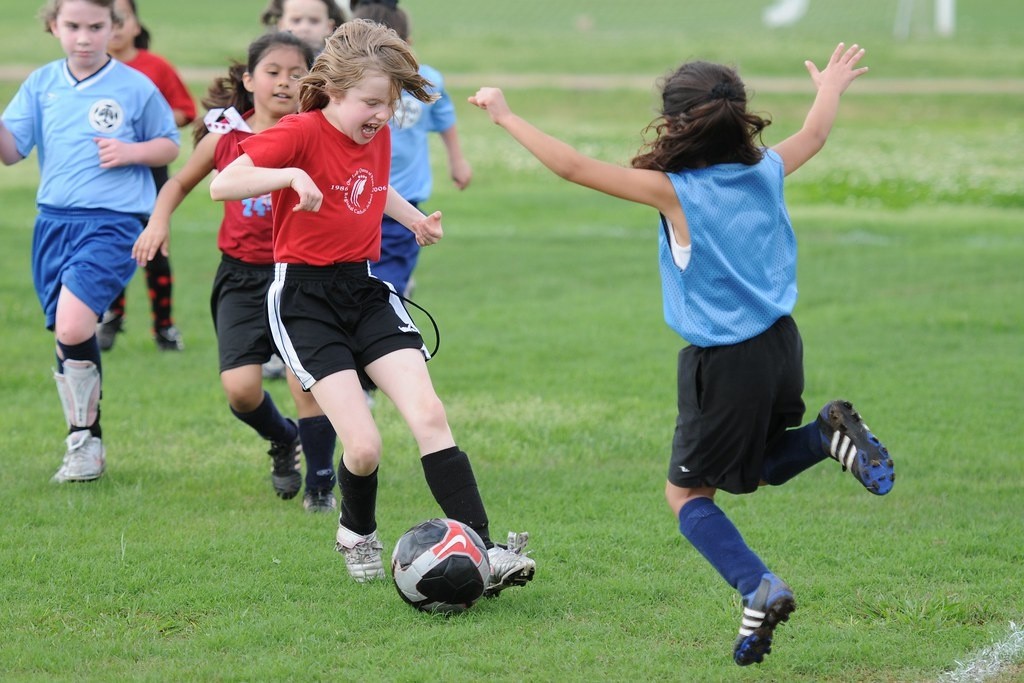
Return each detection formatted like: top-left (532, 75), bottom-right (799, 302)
top-left (483, 531), bottom-right (536, 599)
top-left (261, 353), bottom-right (287, 378)
top-left (817, 399), bottom-right (896, 495)
top-left (333, 512), bottom-right (385, 583)
top-left (48, 430), bottom-right (105, 485)
top-left (267, 418), bottom-right (302, 499)
top-left (734, 573), bottom-right (795, 666)
top-left (302, 489), bottom-right (337, 515)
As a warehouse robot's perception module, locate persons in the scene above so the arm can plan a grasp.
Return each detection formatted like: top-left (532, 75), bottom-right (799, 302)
top-left (466, 41), bottom-right (896, 665)
top-left (0, 2), bottom-right (536, 600)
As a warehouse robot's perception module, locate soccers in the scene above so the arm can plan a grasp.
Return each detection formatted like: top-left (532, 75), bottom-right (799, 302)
top-left (389, 516), bottom-right (493, 616)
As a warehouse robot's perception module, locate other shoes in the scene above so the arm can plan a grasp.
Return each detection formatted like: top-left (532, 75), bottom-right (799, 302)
top-left (153, 326), bottom-right (183, 350)
top-left (402, 278), bottom-right (415, 310)
top-left (363, 388), bottom-right (377, 416)
top-left (97, 311), bottom-right (123, 351)
